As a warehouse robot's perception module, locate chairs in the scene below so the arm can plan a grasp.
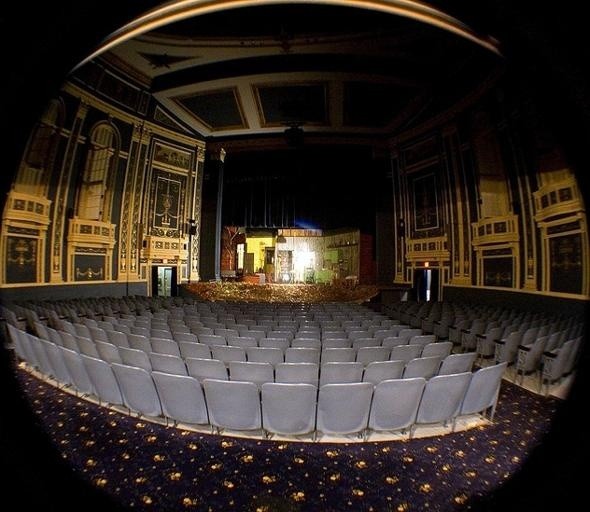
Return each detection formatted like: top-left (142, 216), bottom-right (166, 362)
top-left (0, 295), bottom-right (590, 445)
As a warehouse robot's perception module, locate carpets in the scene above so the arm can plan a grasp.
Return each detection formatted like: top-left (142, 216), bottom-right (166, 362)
top-left (0, 347), bottom-right (590, 512)
